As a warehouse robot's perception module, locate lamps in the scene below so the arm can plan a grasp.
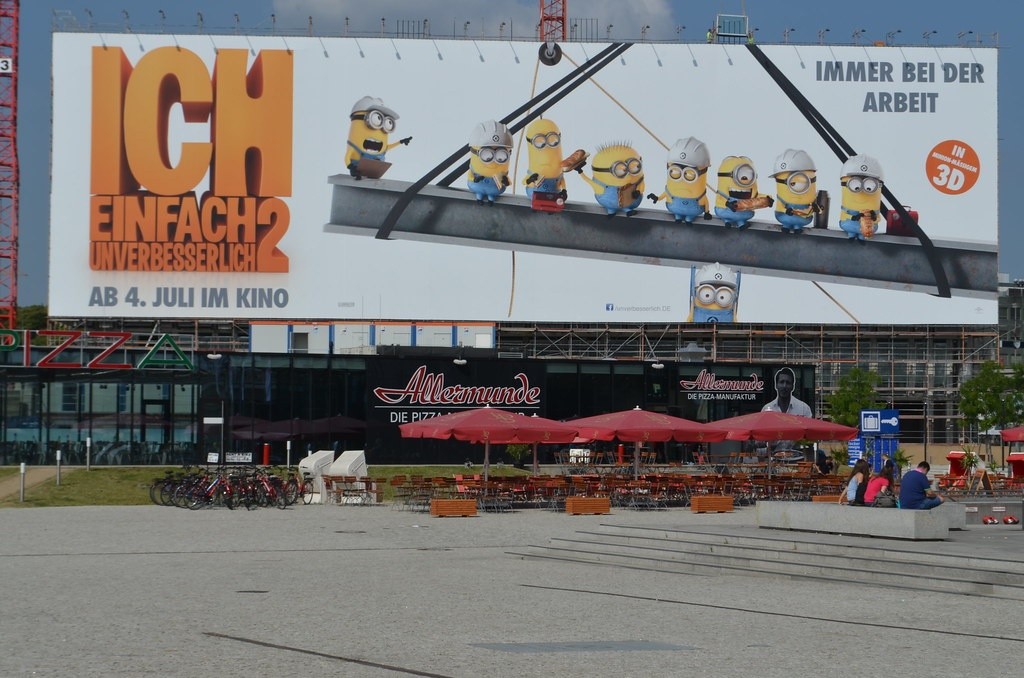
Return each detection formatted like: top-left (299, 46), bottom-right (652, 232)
top-left (53, 8), bottom-right (973, 46)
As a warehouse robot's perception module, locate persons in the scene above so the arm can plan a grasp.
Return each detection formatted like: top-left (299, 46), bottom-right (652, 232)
top-left (813, 449), bottom-right (834, 476)
top-left (697, 444), bottom-right (704, 466)
top-left (762, 368), bottom-right (812, 418)
top-left (839, 454), bottom-right (899, 508)
top-left (899, 462), bottom-right (945, 509)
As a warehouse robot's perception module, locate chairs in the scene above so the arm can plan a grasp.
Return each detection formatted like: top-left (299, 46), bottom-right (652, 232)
top-left (322, 450), bottom-right (1024, 513)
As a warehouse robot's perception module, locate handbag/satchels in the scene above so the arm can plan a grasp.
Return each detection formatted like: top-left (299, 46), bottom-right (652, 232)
top-left (874, 492), bottom-right (898, 508)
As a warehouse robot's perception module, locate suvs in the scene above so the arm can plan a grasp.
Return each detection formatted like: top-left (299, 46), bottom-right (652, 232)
top-left (773, 448), bottom-right (833, 476)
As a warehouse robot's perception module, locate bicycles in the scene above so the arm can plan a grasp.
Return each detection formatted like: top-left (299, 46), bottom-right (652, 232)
top-left (149, 462), bottom-right (315, 510)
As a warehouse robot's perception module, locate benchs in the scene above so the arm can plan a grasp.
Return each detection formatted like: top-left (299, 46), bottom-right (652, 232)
top-left (754, 501), bottom-right (949, 540)
top-left (931, 502), bottom-right (966, 531)
top-left (430, 500), bottom-right (479, 517)
top-left (812, 496), bottom-right (847, 502)
top-left (565, 497), bottom-right (610, 515)
top-left (690, 496), bottom-right (733, 513)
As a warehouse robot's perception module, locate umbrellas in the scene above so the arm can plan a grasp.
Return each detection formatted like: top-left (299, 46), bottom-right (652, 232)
top-left (567, 405), bottom-right (711, 496)
top-left (705, 410), bottom-right (858, 493)
top-left (399, 403), bottom-right (576, 501)
top-left (1000, 426), bottom-right (1024, 442)
top-left (187, 413), bottom-right (370, 444)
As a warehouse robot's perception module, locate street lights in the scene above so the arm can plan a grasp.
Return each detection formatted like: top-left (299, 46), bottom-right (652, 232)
top-left (924, 401), bottom-right (927, 461)
top-left (1001, 398), bottom-right (1005, 470)
